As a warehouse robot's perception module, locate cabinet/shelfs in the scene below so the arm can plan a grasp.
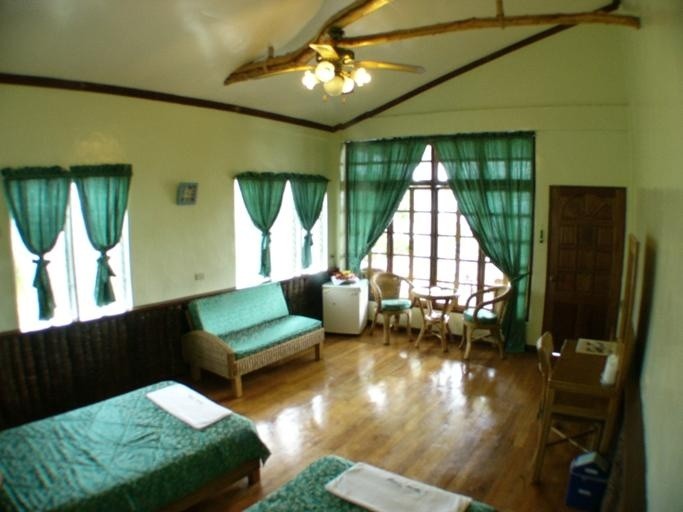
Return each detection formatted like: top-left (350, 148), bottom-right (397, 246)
top-left (321, 278), bottom-right (369, 336)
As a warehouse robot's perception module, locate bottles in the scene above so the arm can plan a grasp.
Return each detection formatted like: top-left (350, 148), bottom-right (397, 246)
top-left (600, 354), bottom-right (618, 385)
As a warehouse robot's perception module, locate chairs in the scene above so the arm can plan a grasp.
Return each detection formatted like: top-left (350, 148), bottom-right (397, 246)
top-left (536, 330), bottom-right (608, 425)
top-left (370, 272), bottom-right (417, 345)
top-left (457, 285), bottom-right (512, 359)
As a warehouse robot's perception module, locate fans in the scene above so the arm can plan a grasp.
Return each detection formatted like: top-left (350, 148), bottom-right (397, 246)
top-left (253, 25), bottom-right (425, 74)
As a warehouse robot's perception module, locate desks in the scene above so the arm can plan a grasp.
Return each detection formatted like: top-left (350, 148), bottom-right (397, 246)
top-left (411, 286), bottom-right (461, 353)
top-left (531, 340), bottom-right (631, 487)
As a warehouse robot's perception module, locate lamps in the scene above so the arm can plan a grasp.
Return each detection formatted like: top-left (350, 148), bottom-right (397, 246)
top-left (301, 60), bottom-right (372, 103)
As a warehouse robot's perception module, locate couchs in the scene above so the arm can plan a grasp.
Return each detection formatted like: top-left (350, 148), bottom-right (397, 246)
top-left (181, 281), bottom-right (325, 398)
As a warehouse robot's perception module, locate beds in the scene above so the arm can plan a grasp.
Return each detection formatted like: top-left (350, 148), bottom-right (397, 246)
top-left (234, 453), bottom-right (495, 512)
top-left (0, 379), bottom-right (274, 512)
